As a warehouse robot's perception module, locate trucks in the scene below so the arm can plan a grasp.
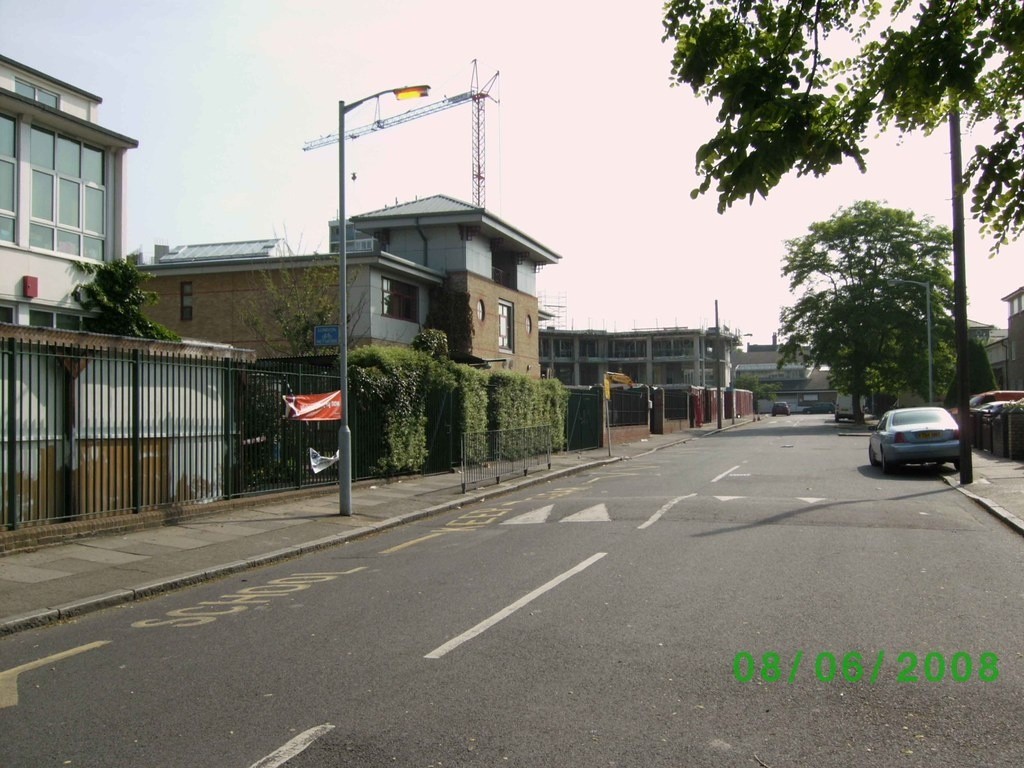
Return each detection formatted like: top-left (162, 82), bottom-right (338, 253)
top-left (835, 391), bottom-right (866, 423)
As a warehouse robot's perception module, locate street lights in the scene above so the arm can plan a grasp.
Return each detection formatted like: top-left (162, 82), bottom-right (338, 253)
top-left (887, 278), bottom-right (934, 406)
top-left (335, 83), bottom-right (432, 517)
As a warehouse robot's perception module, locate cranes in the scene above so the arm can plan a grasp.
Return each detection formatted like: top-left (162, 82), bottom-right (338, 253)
top-left (300, 59), bottom-right (504, 206)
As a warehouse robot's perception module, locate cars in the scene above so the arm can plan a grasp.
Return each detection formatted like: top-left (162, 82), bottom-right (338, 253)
top-left (969, 391), bottom-right (1024, 408)
top-left (772, 402), bottom-right (791, 416)
top-left (869, 407), bottom-right (960, 472)
top-left (803, 402), bottom-right (836, 414)
